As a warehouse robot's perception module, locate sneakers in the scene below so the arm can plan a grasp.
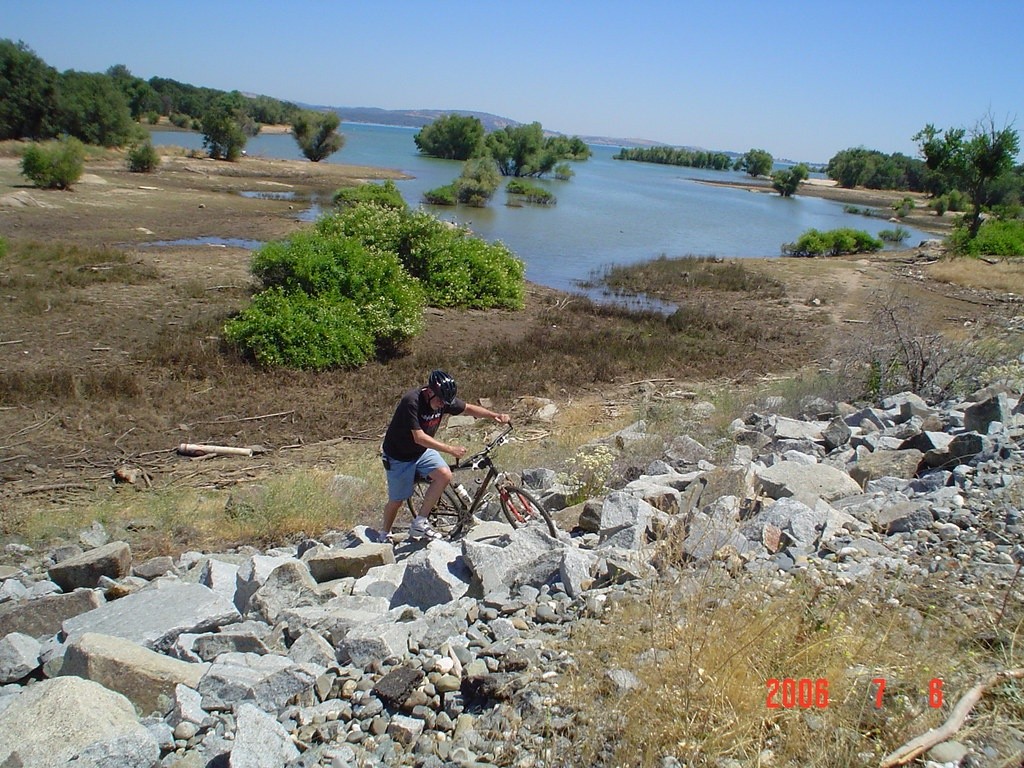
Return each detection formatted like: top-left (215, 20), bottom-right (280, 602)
top-left (376, 536), bottom-right (391, 545)
top-left (408, 521), bottom-right (442, 540)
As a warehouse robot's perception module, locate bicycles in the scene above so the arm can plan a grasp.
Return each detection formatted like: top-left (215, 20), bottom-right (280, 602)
top-left (408, 419), bottom-right (558, 541)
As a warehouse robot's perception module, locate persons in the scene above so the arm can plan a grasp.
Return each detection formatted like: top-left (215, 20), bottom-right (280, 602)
top-left (378, 370), bottom-right (511, 544)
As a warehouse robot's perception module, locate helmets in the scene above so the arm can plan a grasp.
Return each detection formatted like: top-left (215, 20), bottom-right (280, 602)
top-left (429, 370), bottom-right (457, 405)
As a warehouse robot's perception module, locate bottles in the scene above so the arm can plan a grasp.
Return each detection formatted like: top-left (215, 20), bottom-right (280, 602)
top-left (453, 482), bottom-right (472, 506)
